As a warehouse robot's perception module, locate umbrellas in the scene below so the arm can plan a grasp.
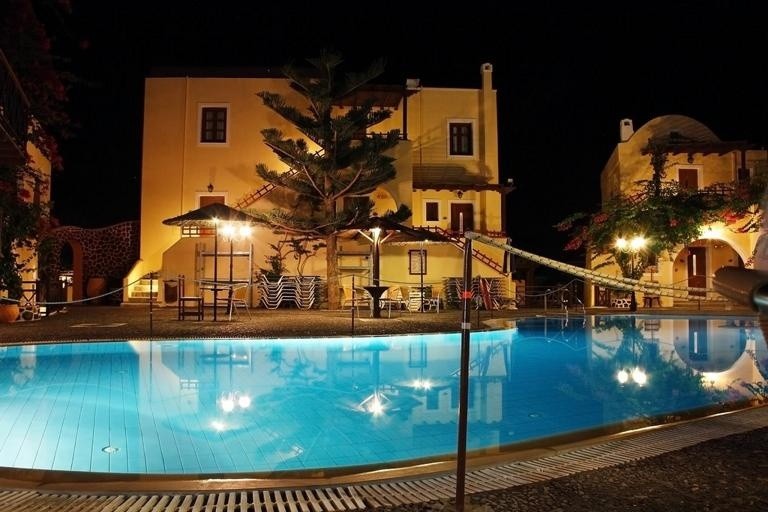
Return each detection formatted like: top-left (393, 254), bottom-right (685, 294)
top-left (384, 228), bottom-right (459, 313)
top-left (315, 216), bottom-right (426, 315)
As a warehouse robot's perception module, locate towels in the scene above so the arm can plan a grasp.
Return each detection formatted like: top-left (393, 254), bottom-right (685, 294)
top-left (163, 282), bottom-right (178, 304)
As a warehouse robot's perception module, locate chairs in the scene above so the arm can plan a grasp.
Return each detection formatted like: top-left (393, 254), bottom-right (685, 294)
top-left (177, 273), bottom-right (205, 322)
top-left (342, 278), bottom-right (524, 316)
top-left (229, 281), bottom-right (253, 322)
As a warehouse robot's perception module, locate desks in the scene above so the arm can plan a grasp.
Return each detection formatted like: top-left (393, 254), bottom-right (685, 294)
top-left (199, 286), bottom-right (236, 322)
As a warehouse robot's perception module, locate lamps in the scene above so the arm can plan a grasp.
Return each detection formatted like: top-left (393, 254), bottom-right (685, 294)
top-left (208, 184), bottom-right (213, 192)
top-left (507, 177), bottom-right (514, 186)
top-left (457, 190), bottom-right (463, 199)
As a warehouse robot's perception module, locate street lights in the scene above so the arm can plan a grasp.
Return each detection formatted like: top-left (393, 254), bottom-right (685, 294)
top-left (336, 215), bottom-right (417, 319)
top-left (332, 339), bottom-right (422, 419)
top-left (217, 344), bottom-right (251, 413)
top-left (218, 224), bottom-right (253, 316)
top-left (615, 316), bottom-right (648, 391)
top-left (163, 340), bottom-right (270, 442)
top-left (615, 229), bottom-right (652, 312)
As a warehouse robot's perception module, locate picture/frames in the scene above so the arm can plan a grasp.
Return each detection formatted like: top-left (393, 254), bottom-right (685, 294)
top-left (408, 342), bottom-right (427, 368)
top-left (408, 249), bottom-right (428, 275)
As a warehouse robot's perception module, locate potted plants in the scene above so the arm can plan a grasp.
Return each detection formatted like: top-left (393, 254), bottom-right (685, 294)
top-left (0, 296), bottom-right (19, 323)
